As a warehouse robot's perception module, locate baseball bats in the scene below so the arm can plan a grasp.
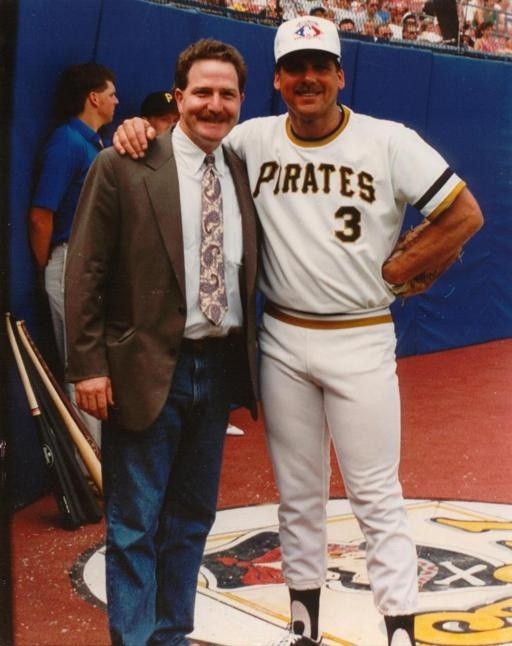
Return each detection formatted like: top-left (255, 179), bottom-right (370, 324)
top-left (5, 311), bottom-right (103, 530)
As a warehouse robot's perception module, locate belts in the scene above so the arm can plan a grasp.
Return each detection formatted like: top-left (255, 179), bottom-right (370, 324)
top-left (180, 336), bottom-right (230, 359)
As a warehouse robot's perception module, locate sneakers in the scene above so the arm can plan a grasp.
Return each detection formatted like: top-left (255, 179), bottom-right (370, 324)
top-left (266, 632), bottom-right (324, 645)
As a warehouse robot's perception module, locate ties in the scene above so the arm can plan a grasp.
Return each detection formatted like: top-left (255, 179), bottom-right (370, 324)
top-left (199, 153), bottom-right (229, 327)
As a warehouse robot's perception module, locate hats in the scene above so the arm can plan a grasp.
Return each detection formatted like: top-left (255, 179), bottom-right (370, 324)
top-left (141, 90), bottom-right (177, 115)
top-left (274, 15), bottom-right (342, 65)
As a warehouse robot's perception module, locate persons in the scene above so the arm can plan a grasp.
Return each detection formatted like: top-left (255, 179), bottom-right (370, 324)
top-left (109, 14), bottom-right (488, 646)
top-left (26, 65), bottom-right (122, 502)
top-left (225, 1), bottom-right (512, 53)
top-left (139, 89), bottom-right (180, 140)
top-left (60, 32), bottom-right (261, 646)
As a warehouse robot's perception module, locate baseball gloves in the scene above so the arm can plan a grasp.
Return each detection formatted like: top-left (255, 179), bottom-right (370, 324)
top-left (384, 217), bottom-right (461, 296)
